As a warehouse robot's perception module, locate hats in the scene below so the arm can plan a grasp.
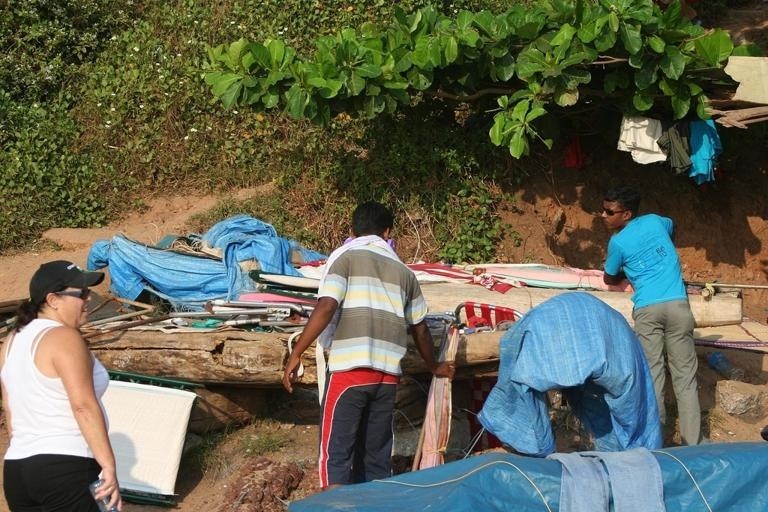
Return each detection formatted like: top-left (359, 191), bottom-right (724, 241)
top-left (30, 260), bottom-right (104, 305)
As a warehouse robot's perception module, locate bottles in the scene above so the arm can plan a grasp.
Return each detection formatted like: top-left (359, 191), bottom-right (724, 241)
top-left (701, 350), bottom-right (745, 383)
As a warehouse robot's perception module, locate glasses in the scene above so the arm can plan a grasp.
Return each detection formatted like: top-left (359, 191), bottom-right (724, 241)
top-left (54, 289), bottom-right (90, 299)
top-left (601, 207), bottom-right (624, 215)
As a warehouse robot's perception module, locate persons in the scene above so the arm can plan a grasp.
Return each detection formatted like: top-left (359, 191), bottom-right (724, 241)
top-left (282, 201), bottom-right (458, 490)
top-left (601, 186), bottom-right (703, 446)
top-left (0, 260), bottom-right (122, 512)
